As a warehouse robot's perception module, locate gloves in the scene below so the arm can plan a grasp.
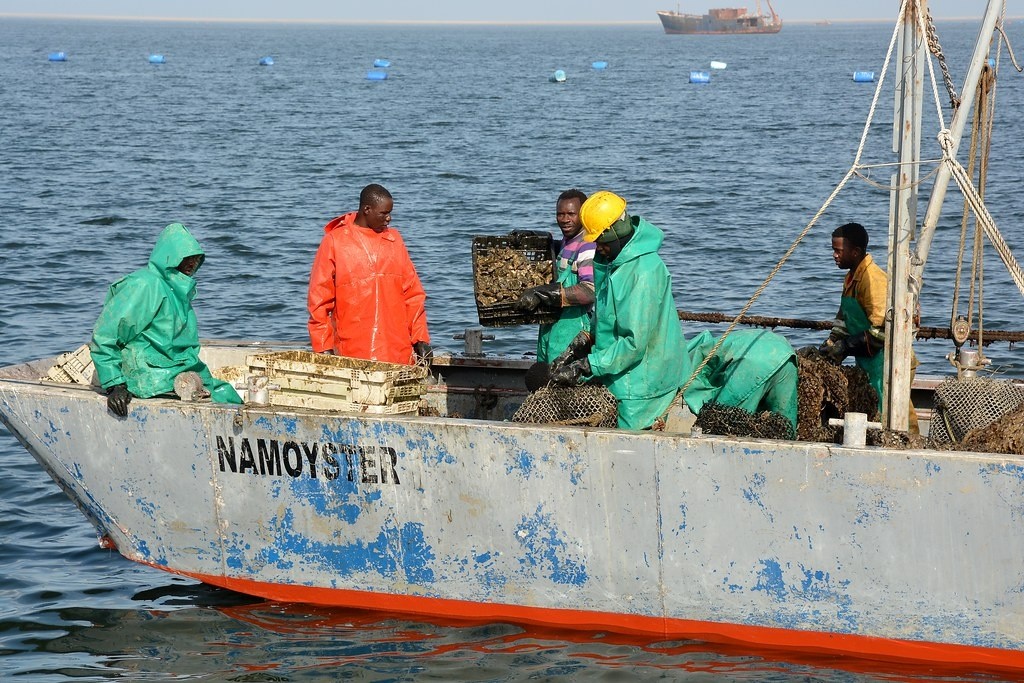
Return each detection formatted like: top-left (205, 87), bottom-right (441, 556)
top-left (320, 349), bottom-right (335, 355)
top-left (819, 339), bottom-right (849, 366)
top-left (508, 230), bottom-right (550, 247)
top-left (412, 341), bottom-right (434, 373)
top-left (553, 357), bottom-right (593, 387)
top-left (106, 384), bottom-right (132, 416)
top-left (514, 282), bottom-right (562, 315)
top-left (548, 330), bottom-right (592, 377)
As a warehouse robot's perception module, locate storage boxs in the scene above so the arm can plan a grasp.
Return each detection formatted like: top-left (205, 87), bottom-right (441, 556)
top-left (40, 343), bottom-right (103, 389)
top-left (471, 233), bottom-right (565, 329)
top-left (247, 350), bottom-right (427, 424)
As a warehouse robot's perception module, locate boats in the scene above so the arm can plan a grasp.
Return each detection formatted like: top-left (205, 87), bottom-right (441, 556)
top-left (0, 1), bottom-right (1024, 683)
top-left (656, 1), bottom-right (782, 34)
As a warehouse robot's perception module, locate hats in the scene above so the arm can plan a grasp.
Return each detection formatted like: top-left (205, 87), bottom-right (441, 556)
top-left (579, 191), bottom-right (627, 242)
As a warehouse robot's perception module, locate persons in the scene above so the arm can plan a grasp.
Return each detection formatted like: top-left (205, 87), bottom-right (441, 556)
top-left (518, 189), bottom-right (695, 430)
top-left (307, 184), bottom-right (434, 372)
top-left (680, 328), bottom-right (800, 439)
top-left (90, 224), bottom-right (245, 417)
top-left (819, 223), bottom-right (921, 433)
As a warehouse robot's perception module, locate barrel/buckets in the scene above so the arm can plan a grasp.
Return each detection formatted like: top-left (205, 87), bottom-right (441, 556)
top-left (689, 70), bottom-right (710, 83)
top-left (989, 58), bottom-right (996, 68)
top-left (591, 61), bottom-right (608, 69)
top-left (710, 60), bottom-right (727, 70)
top-left (852, 71), bottom-right (875, 82)
top-left (548, 70), bottom-right (566, 82)
